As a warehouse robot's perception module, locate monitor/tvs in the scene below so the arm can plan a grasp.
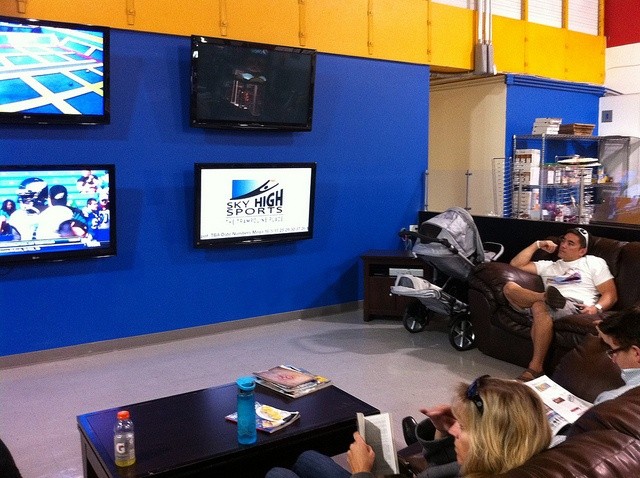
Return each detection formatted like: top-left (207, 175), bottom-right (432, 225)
top-left (194, 163), bottom-right (316, 248)
top-left (189, 35), bottom-right (316, 131)
top-left (0, 16), bottom-right (110, 126)
top-left (0, 164), bottom-right (116, 266)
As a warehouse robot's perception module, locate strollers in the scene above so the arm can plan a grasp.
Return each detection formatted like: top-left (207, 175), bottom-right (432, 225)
top-left (387, 207), bottom-right (504, 353)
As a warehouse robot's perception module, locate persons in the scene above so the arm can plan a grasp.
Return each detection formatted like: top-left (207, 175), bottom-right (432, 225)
top-left (594, 303), bottom-right (640, 405)
top-left (0, 169), bottom-right (111, 253)
top-left (265, 375), bottom-right (552, 477)
top-left (503, 227), bottom-right (619, 382)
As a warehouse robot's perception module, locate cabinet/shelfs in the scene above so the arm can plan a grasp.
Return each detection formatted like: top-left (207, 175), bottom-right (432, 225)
top-left (511, 134), bottom-right (629, 222)
top-left (362, 248), bottom-right (434, 322)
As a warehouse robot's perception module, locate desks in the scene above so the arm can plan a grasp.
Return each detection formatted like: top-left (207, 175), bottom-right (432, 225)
top-left (76, 380), bottom-right (380, 478)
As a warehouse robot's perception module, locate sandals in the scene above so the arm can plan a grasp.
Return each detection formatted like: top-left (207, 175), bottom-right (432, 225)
top-left (544, 285), bottom-right (566, 312)
top-left (516, 368), bottom-right (545, 382)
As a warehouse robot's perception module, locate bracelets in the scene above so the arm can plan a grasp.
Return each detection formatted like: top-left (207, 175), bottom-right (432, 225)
top-left (595, 304), bottom-right (603, 314)
top-left (537, 240), bottom-right (540, 249)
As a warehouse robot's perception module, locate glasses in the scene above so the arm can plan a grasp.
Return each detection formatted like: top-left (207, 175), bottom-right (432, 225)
top-left (606, 346), bottom-right (627, 359)
top-left (576, 226), bottom-right (589, 249)
top-left (466, 375), bottom-right (491, 415)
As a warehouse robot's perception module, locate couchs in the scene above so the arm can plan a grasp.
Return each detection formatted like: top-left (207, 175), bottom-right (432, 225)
top-left (500, 332), bottom-right (639, 477)
top-left (470, 233), bottom-right (640, 372)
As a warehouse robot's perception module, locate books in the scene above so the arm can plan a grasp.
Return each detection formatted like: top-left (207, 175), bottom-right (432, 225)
top-left (253, 363), bottom-right (334, 399)
top-left (356, 411), bottom-right (400, 477)
top-left (522, 374), bottom-right (594, 439)
top-left (224, 400), bottom-right (301, 434)
top-left (513, 187), bottom-right (539, 220)
top-left (531, 117), bottom-right (596, 137)
top-left (513, 148), bottom-right (541, 185)
top-left (545, 163), bottom-right (592, 186)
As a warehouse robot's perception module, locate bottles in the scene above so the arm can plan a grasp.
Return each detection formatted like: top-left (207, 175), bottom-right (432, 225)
top-left (235, 375), bottom-right (257, 444)
top-left (114, 411), bottom-right (137, 467)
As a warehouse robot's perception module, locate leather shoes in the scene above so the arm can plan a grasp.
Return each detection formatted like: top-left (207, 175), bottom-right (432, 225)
top-left (402, 416), bottom-right (418, 446)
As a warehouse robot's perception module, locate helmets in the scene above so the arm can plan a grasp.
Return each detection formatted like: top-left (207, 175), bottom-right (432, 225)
top-left (15, 177), bottom-right (48, 214)
